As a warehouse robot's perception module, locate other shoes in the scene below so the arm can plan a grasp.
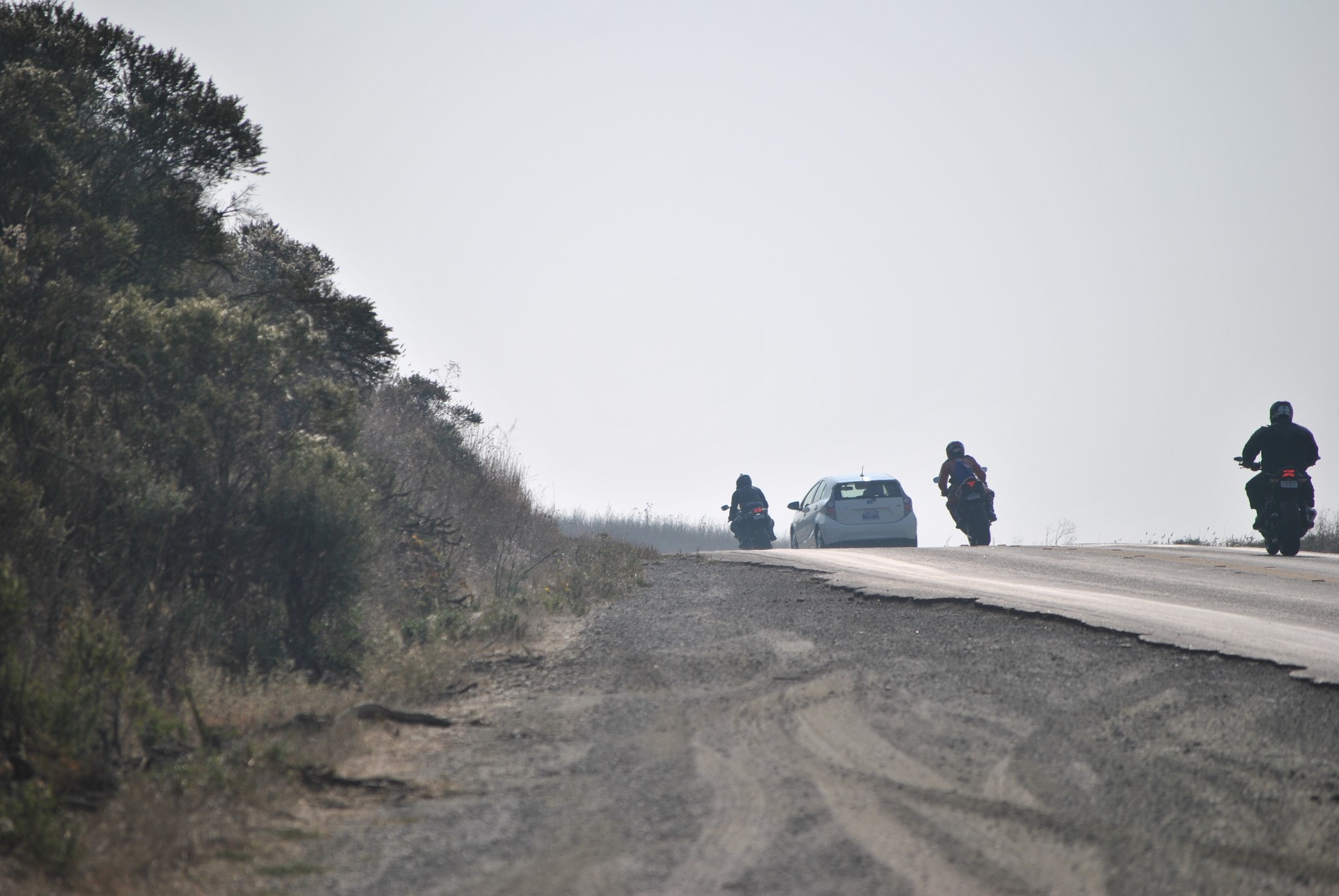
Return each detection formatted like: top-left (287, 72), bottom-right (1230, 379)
top-left (1253, 516), bottom-right (1267, 530)
top-left (770, 534), bottom-right (776, 540)
top-left (988, 512), bottom-right (997, 521)
top-left (738, 541), bottom-right (749, 548)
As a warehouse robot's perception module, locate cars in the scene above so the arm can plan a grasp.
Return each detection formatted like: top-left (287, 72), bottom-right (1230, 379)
top-left (787, 473), bottom-right (917, 549)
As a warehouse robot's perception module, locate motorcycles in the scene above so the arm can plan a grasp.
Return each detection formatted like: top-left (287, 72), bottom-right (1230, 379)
top-left (933, 467), bottom-right (995, 546)
top-left (1234, 456), bottom-right (1322, 557)
top-left (720, 504), bottom-right (773, 550)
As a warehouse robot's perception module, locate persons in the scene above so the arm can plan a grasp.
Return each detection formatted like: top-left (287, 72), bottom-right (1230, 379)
top-left (729, 475), bottom-right (777, 548)
top-left (1243, 401), bottom-right (1315, 530)
top-left (938, 441), bottom-right (996, 529)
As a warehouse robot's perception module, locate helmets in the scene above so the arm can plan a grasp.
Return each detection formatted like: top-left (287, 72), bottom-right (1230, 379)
top-left (946, 441), bottom-right (965, 459)
top-left (1270, 401), bottom-right (1293, 423)
top-left (736, 474), bottom-right (752, 489)
